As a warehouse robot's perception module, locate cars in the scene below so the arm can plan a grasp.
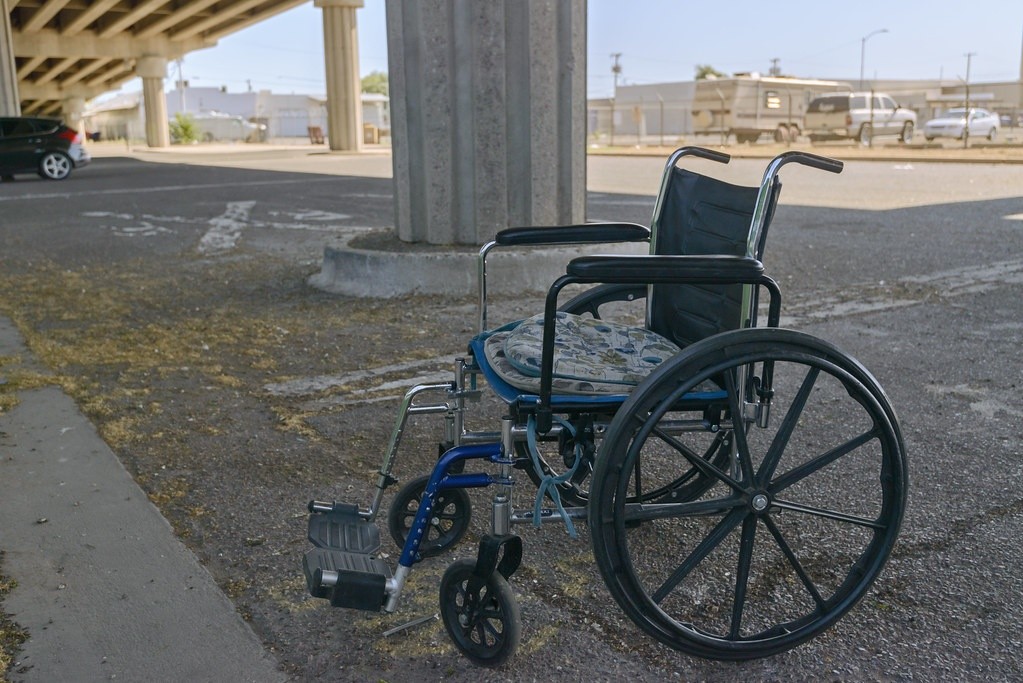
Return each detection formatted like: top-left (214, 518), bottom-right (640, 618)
top-left (923, 107), bottom-right (1000, 143)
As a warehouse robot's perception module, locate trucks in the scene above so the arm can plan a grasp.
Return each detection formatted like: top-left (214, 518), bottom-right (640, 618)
top-left (691, 77), bottom-right (852, 143)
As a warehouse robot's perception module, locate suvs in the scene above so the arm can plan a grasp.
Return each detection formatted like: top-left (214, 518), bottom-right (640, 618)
top-left (0, 116), bottom-right (90, 181)
top-left (804, 91), bottom-right (917, 149)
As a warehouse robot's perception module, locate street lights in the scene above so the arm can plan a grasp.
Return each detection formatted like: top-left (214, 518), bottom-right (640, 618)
top-left (861, 29), bottom-right (889, 90)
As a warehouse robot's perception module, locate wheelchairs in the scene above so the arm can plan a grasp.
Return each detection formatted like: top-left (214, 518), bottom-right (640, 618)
top-left (305, 145), bottom-right (909, 666)
